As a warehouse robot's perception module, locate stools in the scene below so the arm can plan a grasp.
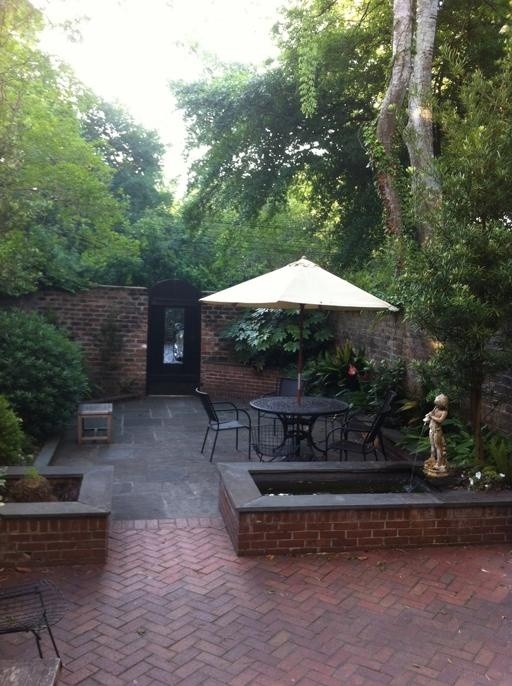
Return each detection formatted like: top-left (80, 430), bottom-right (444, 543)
top-left (76, 401), bottom-right (113, 445)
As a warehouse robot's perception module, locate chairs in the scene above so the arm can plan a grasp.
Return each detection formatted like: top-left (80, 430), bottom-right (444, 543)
top-left (326, 391), bottom-right (396, 461)
top-left (194, 388), bottom-right (252, 461)
top-left (1, 564), bottom-right (67, 668)
top-left (342, 389), bottom-right (401, 462)
top-left (259, 376), bottom-right (310, 435)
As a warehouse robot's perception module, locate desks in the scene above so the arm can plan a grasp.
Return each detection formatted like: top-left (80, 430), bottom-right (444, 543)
top-left (250, 396), bottom-right (348, 460)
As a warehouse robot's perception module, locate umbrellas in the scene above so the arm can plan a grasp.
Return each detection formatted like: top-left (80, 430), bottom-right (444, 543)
top-left (200, 257), bottom-right (403, 406)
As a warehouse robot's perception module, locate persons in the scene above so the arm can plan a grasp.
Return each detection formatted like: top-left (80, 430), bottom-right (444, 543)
top-left (421, 392), bottom-right (448, 470)
